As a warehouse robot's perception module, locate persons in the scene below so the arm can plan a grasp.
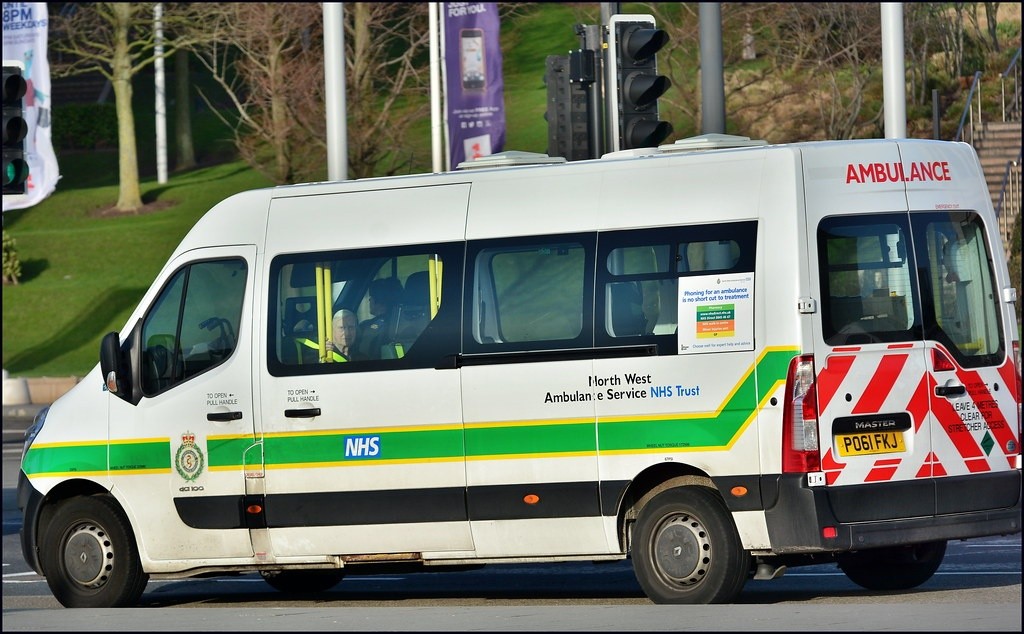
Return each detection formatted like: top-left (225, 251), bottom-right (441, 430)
top-left (326, 309), bottom-right (370, 363)
top-left (359, 278), bottom-right (405, 343)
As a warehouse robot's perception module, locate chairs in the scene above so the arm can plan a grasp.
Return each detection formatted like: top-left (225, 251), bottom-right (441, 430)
top-left (282, 296), bottom-right (318, 362)
top-left (610, 282), bottom-right (645, 334)
top-left (369, 270), bottom-right (431, 361)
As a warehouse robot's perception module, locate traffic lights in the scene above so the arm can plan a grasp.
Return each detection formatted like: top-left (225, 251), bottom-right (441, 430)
top-left (615, 21), bottom-right (673, 152)
top-left (0, 66), bottom-right (29, 196)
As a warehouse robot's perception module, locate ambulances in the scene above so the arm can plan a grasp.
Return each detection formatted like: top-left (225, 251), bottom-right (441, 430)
top-left (18, 133), bottom-right (1024, 609)
